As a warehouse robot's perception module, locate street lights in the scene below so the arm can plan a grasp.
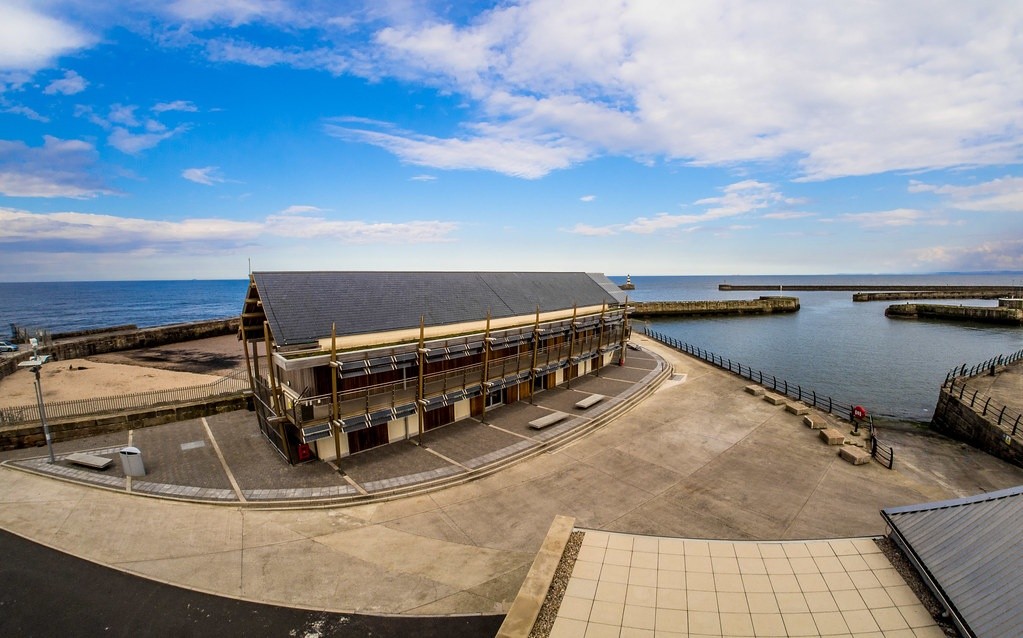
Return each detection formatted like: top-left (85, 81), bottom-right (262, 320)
top-left (18, 337), bottom-right (55, 464)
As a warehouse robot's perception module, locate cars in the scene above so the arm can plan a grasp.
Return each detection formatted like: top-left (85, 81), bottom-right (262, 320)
top-left (0, 340), bottom-right (18, 352)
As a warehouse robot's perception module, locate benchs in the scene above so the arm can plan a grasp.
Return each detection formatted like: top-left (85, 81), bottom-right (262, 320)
top-left (764, 392), bottom-right (786, 406)
top-left (839, 445), bottom-right (871, 466)
top-left (746, 384), bottom-right (765, 396)
top-left (819, 428), bottom-right (844, 447)
top-left (528, 411), bottom-right (571, 429)
top-left (804, 414), bottom-right (827, 428)
top-left (786, 402), bottom-right (809, 415)
top-left (576, 394), bottom-right (604, 408)
top-left (65, 453), bottom-right (113, 468)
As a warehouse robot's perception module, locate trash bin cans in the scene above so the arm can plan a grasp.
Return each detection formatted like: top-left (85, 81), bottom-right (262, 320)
top-left (119, 447), bottom-right (147, 477)
